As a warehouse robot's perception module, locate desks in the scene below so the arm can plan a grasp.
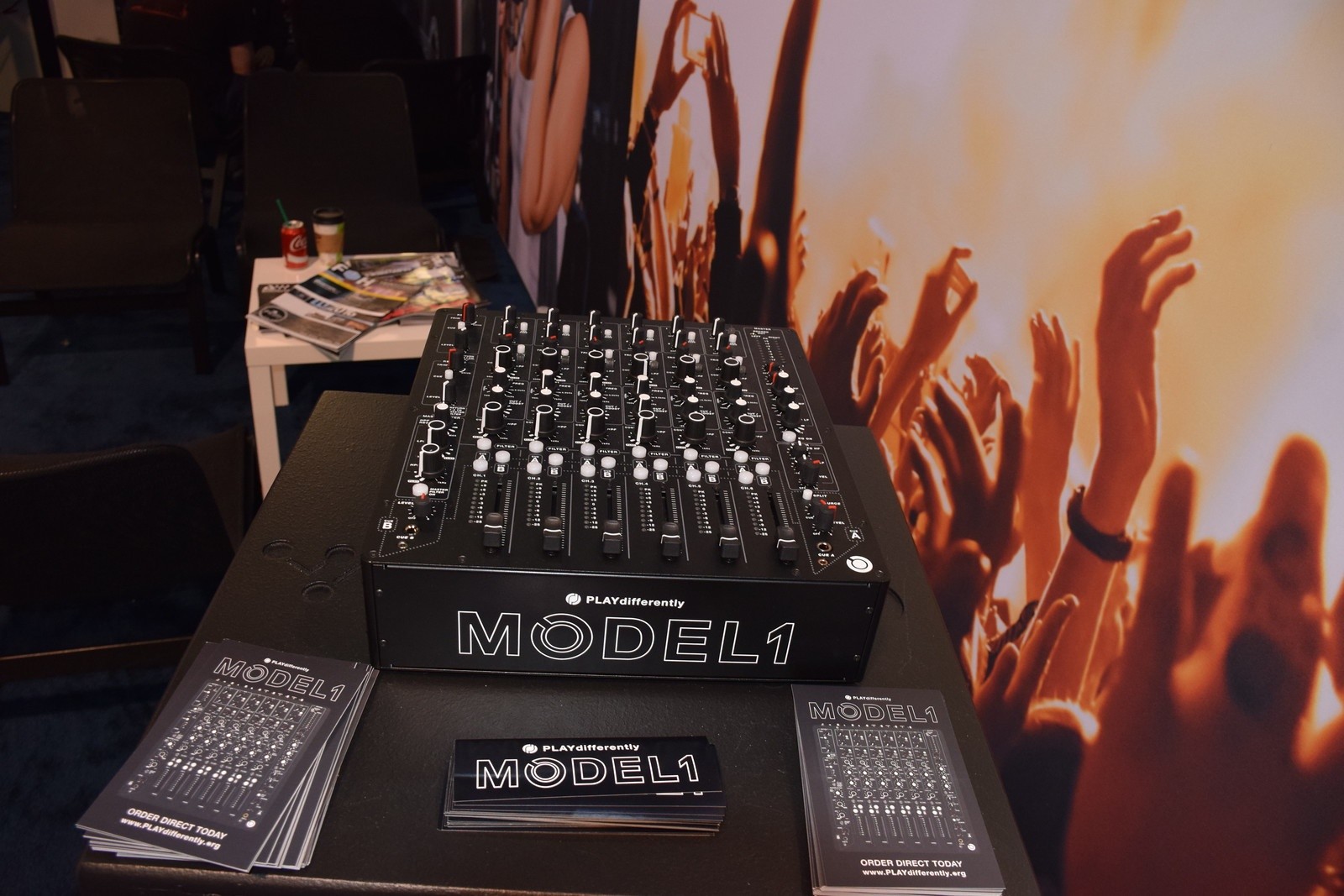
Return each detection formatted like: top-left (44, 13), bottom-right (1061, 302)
top-left (70, 391), bottom-right (1044, 896)
top-left (244, 252), bottom-right (479, 503)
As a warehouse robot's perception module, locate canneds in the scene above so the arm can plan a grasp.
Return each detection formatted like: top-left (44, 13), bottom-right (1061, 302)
top-left (281, 220), bottom-right (308, 269)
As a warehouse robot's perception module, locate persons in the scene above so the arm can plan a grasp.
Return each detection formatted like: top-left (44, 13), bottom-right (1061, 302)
top-left (492, 1), bottom-right (1343, 895)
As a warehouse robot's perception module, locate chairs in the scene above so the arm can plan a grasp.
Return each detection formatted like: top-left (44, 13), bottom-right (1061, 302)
top-left (1, 76), bottom-right (223, 377)
top-left (235, 71), bottom-right (442, 266)
top-left (0, 419), bottom-right (256, 684)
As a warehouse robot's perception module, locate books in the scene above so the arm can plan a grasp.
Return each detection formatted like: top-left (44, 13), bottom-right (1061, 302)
top-left (245, 251), bottom-right (480, 354)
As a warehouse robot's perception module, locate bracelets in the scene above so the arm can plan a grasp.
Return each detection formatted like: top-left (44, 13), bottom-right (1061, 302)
top-left (1066, 483), bottom-right (1133, 561)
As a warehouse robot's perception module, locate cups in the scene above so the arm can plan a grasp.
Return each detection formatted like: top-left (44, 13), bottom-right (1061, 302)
top-left (312, 207), bottom-right (346, 267)
